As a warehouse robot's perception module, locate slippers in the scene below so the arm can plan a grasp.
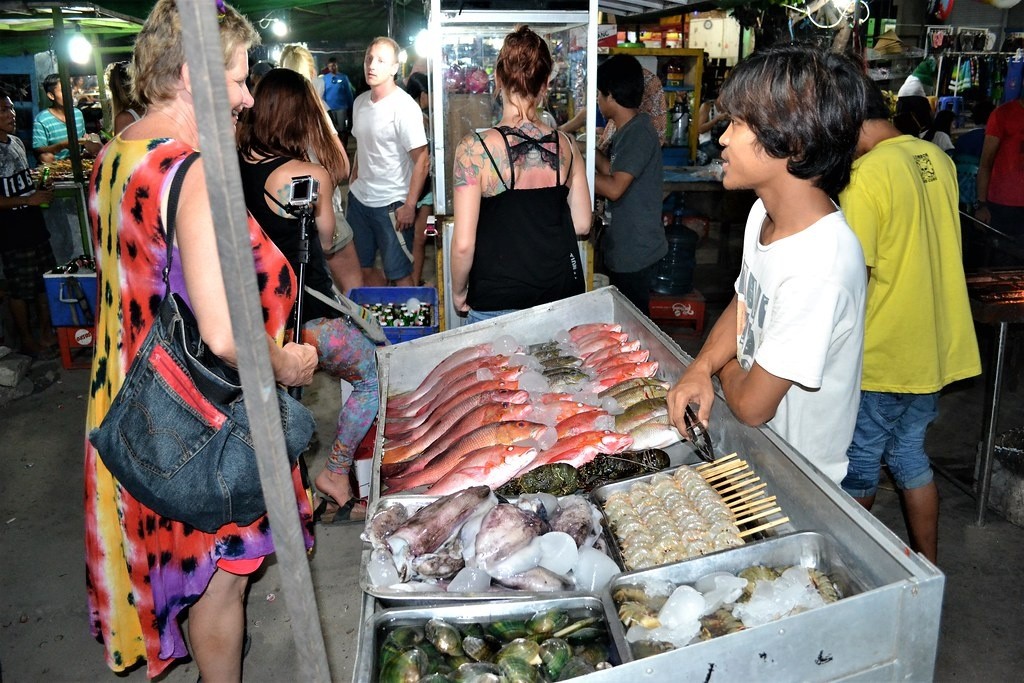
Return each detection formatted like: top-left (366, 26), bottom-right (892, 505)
top-left (313, 499), bottom-right (339, 524)
top-left (322, 498), bottom-right (368, 524)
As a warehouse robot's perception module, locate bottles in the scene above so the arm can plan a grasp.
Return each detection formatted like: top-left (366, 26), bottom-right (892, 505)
top-left (446, 44), bottom-right (498, 93)
top-left (53, 255), bottom-right (96, 274)
top-left (651, 209), bottom-right (699, 294)
top-left (362, 303), bottom-right (431, 326)
top-left (39, 168), bottom-right (50, 207)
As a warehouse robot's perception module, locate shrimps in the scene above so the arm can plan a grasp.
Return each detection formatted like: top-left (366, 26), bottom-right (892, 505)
top-left (606, 464), bottom-right (745, 573)
top-left (608, 552), bottom-right (844, 659)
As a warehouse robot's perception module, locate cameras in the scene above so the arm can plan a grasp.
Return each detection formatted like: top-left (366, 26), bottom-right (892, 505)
top-left (289, 176), bottom-right (320, 206)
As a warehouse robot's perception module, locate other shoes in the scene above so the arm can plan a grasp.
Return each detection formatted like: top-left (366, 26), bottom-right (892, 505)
top-left (38, 336), bottom-right (59, 348)
top-left (22, 344), bottom-right (49, 354)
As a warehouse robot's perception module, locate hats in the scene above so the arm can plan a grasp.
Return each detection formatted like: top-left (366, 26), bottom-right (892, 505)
top-left (897, 74), bottom-right (926, 98)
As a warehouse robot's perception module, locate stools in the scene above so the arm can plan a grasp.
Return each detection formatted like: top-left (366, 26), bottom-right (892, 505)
top-left (57, 325), bottom-right (95, 369)
top-left (650, 292), bottom-right (705, 334)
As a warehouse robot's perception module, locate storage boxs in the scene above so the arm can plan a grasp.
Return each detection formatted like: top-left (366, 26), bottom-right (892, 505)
top-left (346, 287), bottom-right (439, 345)
top-left (43, 271), bottom-right (99, 327)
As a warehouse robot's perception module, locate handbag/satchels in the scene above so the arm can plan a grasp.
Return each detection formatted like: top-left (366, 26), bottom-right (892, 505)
top-left (342, 298), bottom-right (387, 343)
top-left (89, 153), bottom-right (317, 532)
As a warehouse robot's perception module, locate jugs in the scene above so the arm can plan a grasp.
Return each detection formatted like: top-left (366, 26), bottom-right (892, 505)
top-left (670, 103), bottom-right (689, 146)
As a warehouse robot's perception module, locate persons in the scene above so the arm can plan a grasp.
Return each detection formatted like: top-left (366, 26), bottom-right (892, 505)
top-left (594, 52), bottom-right (671, 318)
top-left (547, 95), bottom-right (606, 153)
top-left (85, 0), bottom-right (318, 683)
top-left (0, 37), bottom-right (428, 352)
top-left (236, 69), bottom-right (380, 524)
top-left (666, 36), bottom-right (872, 490)
top-left (599, 61), bottom-right (667, 157)
top-left (841, 70), bottom-right (983, 564)
top-left (450, 26), bottom-right (592, 324)
top-left (696, 42), bottom-right (1024, 269)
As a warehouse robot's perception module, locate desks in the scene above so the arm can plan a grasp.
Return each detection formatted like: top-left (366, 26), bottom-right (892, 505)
top-left (662, 165), bottom-right (740, 268)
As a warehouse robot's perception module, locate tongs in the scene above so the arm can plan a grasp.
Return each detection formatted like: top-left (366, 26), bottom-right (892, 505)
top-left (683, 405), bottom-right (715, 462)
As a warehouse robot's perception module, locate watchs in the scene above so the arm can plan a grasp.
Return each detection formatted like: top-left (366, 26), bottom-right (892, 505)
top-left (977, 200), bottom-right (986, 209)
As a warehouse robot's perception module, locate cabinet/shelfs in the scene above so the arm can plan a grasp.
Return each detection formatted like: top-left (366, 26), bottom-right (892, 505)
top-left (596, 45), bottom-right (705, 165)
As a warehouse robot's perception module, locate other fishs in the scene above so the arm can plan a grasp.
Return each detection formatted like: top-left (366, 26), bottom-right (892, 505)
top-left (363, 484), bottom-right (596, 600)
top-left (379, 319), bottom-right (709, 499)
